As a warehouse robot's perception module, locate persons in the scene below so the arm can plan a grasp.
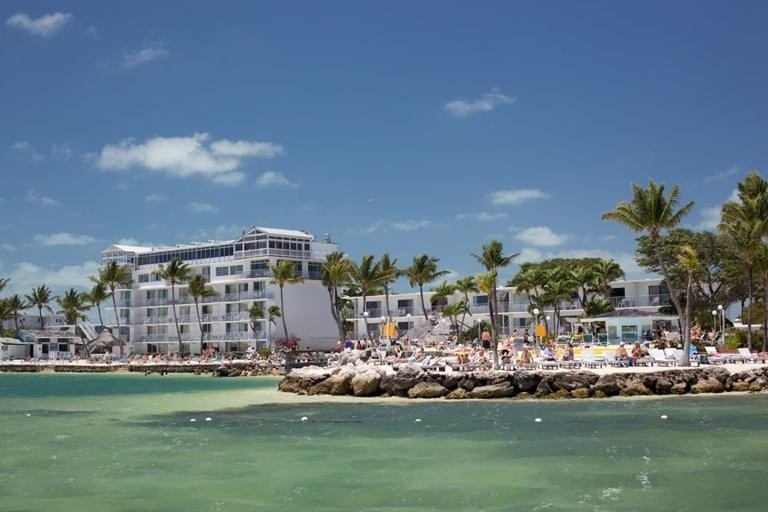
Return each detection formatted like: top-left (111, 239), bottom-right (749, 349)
top-left (335, 341), bottom-right (343, 353)
top-left (426, 329), bottom-right (434, 346)
top-left (515, 347), bottom-right (533, 368)
top-left (248, 347), bottom-right (255, 360)
top-left (612, 341), bottom-right (633, 367)
top-left (344, 336), bottom-right (352, 349)
top-left (256, 351), bottom-right (260, 359)
top-left (480, 327), bottom-right (493, 353)
top-left (441, 331), bottom-right (453, 349)
top-left (393, 347), bottom-right (424, 363)
top-left (522, 329), bottom-right (530, 344)
top-left (543, 345), bottom-right (555, 361)
top-left (630, 341), bottom-right (642, 359)
top-left (282, 343), bottom-right (311, 352)
top-left (561, 341), bottom-right (574, 360)
top-left (511, 329), bottom-right (518, 337)
top-left (477, 351), bottom-right (488, 365)
top-left (471, 336), bottom-right (478, 349)
top-left (356, 340), bottom-right (362, 349)
top-left (245, 345), bottom-right (250, 357)
top-left (362, 338), bottom-right (369, 348)
top-left (208, 345), bottom-right (215, 357)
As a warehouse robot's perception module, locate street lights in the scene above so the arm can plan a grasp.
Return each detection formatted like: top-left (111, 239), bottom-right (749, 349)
top-left (363, 312), bottom-right (369, 349)
top-left (711, 305), bottom-right (725, 333)
top-left (477, 319), bottom-right (481, 341)
top-left (406, 313), bottom-right (412, 341)
top-left (533, 307), bottom-right (540, 348)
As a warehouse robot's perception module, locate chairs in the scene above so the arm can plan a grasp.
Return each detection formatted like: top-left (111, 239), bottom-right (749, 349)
top-left (365, 340), bottom-right (768, 372)
